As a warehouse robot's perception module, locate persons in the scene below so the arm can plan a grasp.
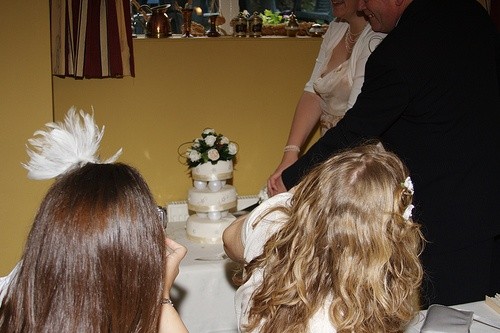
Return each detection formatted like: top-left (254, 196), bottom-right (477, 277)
top-left (0, 162), bottom-right (189, 333)
top-left (269, 0), bottom-right (500, 310)
top-left (267, 0), bottom-right (388, 197)
top-left (223, 146), bottom-right (423, 333)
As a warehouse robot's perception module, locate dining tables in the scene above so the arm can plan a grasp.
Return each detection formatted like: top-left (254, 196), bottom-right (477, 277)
top-left (163, 218), bottom-right (242, 333)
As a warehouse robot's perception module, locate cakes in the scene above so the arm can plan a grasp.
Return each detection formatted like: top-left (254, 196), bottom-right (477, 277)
top-left (186, 128), bottom-right (237, 241)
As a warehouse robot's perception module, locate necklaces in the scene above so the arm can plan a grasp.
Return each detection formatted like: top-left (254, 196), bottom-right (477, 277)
top-left (345, 21), bottom-right (369, 53)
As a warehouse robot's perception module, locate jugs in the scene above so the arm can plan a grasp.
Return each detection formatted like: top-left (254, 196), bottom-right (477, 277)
top-left (138, 4), bottom-right (172, 37)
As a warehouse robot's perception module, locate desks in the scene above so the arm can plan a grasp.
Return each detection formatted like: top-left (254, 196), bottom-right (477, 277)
top-left (405, 300), bottom-right (500, 333)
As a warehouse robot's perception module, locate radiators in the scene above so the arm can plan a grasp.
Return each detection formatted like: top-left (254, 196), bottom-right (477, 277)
top-left (166, 195), bottom-right (257, 225)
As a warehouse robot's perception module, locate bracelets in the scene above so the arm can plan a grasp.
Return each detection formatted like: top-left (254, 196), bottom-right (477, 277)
top-left (160, 297), bottom-right (174, 306)
top-left (284, 145), bottom-right (300, 152)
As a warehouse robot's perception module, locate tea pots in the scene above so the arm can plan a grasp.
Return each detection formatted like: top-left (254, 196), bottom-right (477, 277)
top-left (305, 23), bottom-right (328, 37)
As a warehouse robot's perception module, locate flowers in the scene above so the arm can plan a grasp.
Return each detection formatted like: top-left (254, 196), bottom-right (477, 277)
top-left (402, 177), bottom-right (416, 195)
top-left (403, 204), bottom-right (415, 219)
top-left (186, 125), bottom-right (239, 170)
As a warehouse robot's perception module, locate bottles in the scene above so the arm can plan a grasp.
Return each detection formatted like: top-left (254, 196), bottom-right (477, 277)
top-left (249, 12), bottom-right (262, 37)
top-left (288, 12), bottom-right (298, 37)
top-left (157, 206), bottom-right (167, 229)
top-left (233, 9), bottom-right (247, 37)
